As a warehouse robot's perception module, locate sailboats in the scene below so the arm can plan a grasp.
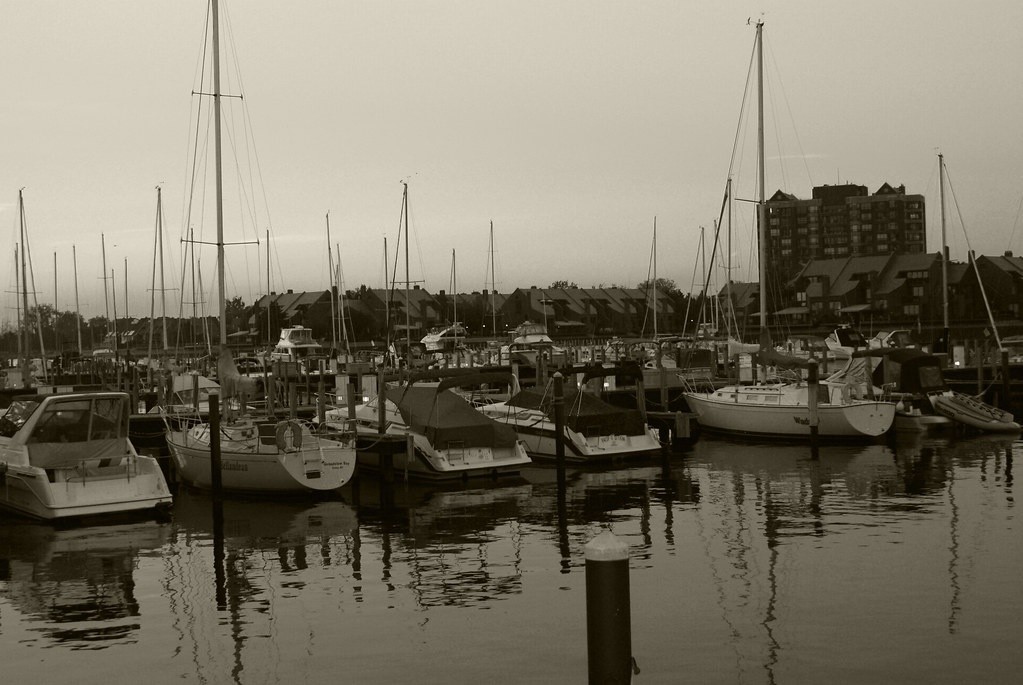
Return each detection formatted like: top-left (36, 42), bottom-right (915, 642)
top-left (0, 3), bottom-right (1023, 496)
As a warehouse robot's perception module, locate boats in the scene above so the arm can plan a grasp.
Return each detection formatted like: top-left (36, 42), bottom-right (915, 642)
top-left (925, 385), bottom-right (1022, 434)
top-left (1, 390), bottom-right (177, 528)
